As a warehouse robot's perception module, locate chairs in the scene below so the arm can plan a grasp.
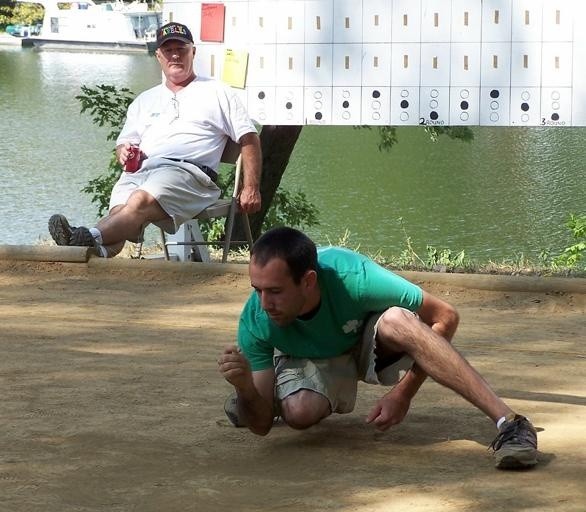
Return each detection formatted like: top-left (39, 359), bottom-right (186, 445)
top-left (160, 136), bottom-right (253, 263)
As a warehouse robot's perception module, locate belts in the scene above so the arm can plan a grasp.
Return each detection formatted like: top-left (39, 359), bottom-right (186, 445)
top-left (164, 157), bottom-right (202, 170)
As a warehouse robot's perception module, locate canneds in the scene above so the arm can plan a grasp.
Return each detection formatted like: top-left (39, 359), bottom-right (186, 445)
top-left (121, 143), bottom-right (140, 172)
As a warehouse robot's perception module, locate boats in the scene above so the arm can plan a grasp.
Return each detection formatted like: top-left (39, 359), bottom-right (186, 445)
top-left (1, 0), bottom-right (163, 51)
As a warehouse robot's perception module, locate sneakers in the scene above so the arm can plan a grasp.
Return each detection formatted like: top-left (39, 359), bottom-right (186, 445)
top-left (69, 227), bottom-right (97, 247)
top-left (48, 214), bottom-right (74, 245)
top-left (223, 392), bottom-right (243, 427)
top-left (487, 412), bottom-right (538, 470)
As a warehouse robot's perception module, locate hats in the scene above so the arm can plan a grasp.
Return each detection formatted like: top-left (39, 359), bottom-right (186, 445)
top-left (155, 22), bottom-right (191, 47)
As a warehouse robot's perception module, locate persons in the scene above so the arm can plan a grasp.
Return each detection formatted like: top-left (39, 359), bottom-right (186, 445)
top-left (47, 22), bottom-right (264, 259)
top-left (215, 226), bottom-right (539, 470)
